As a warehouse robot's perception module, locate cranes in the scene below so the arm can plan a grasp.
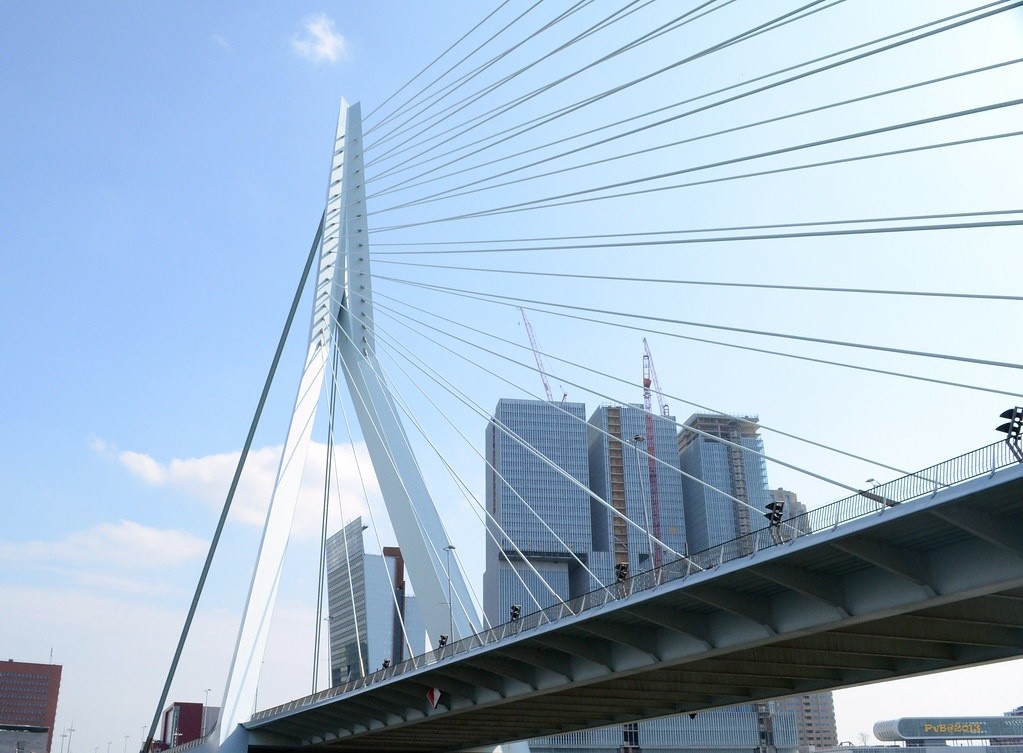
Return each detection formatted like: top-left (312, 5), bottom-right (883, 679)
top-left (516, 304), bottom-right (568, 403)
top-left (641, 336), bottom-right (669, 418)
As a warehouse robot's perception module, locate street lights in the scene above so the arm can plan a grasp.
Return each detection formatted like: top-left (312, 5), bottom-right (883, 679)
top-left (442, 545), bottom-right (456, 656)
top-left (202, 688), bottom-right (212, 736)
top-left (107, 741), bottom-right (112, 753)
top-left (94, 746), bottom-right (100, 753)
top-left (123, 735), bottom-right (129, 753)
top-left (140, 725), bottom-right (148, 744)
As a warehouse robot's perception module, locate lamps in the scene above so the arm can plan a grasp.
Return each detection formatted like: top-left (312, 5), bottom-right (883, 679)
top-left (763, 500), bottom-right (785, 544)
top-left (995, 406), bottom-right (1023, 465)
top-left (510, 604), bottom-right (521, 633)
top-left (437, 634), bottom-right (449, 658)
top-left (383, 659), bottom-right (390, 671)
top-left (614, 562), bottom-right (629, 598)
